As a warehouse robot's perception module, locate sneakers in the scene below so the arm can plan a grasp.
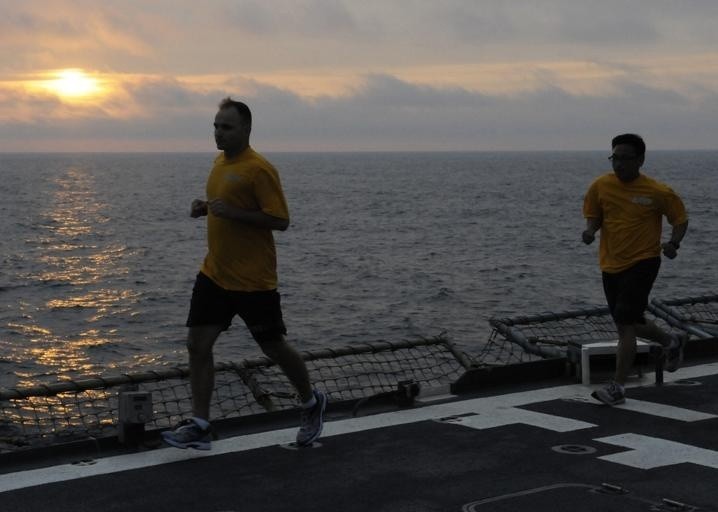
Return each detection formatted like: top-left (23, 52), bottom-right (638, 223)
top-left (664, 328), bottom-right (688, 373)
top-left (294, 388), bottom-right (329, 447)
top-left (157, 416), bottom-right (214, 451)
top-left (591, 380), bottom-right (627, 406)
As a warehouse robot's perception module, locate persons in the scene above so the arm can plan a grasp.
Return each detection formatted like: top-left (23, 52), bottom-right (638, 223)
top-left (160, 97), bottom-right (327, 450)
top-left (582, 134), bottom-right (689, 406)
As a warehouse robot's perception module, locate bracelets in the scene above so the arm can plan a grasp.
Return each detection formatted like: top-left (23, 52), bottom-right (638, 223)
top-left (668, 239), bottom-right (680, 249)
